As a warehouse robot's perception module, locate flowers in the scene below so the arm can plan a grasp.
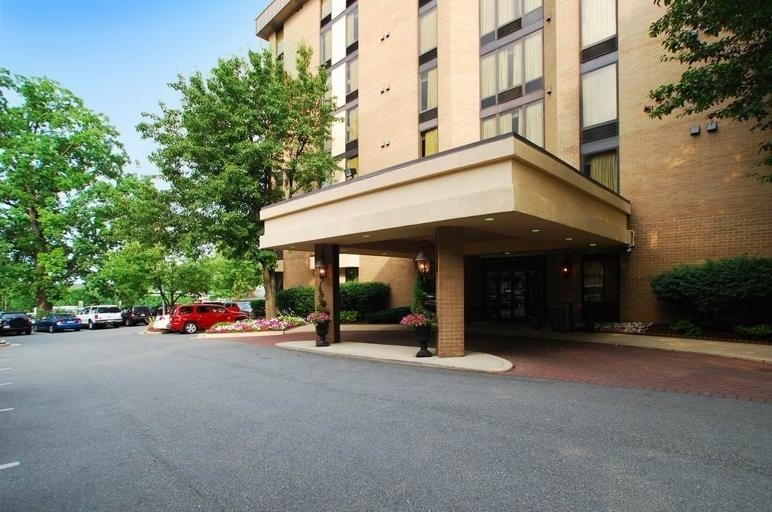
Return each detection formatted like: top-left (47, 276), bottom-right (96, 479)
top-left (399, 312), bottom-right (432, 327)
top-left (304, 310), bottom-right (332, 326)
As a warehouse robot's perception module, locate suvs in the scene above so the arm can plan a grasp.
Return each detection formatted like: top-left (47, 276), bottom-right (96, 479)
top-left (152, 303), bottom-right (176, 316)
top-left (119, 305), bottom-right (155, 326)
top-left (73, 304), bottom-right (123, 329)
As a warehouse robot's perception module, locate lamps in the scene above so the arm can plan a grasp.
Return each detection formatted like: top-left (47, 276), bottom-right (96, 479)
top-left (315, 247), bottom-right (328, 278)
top-left (414, 248), bottom-right (436, 284)
top-left (559, 262), bottom-right (570, 280)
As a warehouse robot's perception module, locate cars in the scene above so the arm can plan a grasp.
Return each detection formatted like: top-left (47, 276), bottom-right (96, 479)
top-left (0, 311), bottom-right (32, 336)
top-left (153, 304), bottom-right (248, 334)
top-left (32, 313), bottom-right (82, 332)
top-left (203, 301), bottom-right (240, 312)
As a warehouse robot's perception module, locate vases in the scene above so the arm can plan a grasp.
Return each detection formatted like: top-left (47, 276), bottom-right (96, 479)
top-left (414, 325), bottom-right (433, 357)
top-left (315, 323), bottom-right (329, 346)
